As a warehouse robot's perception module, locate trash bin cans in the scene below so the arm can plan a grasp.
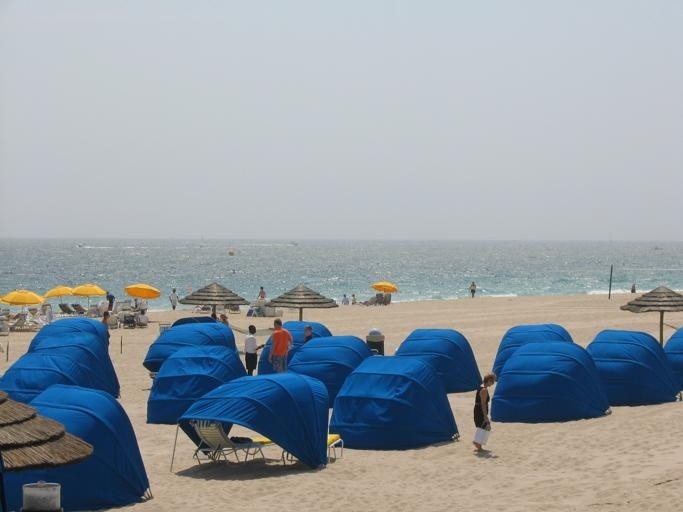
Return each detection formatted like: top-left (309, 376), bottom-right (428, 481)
top-left (366, 328), bottom-right (385, 355)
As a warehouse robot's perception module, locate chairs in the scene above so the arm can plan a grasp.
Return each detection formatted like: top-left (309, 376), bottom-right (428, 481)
top-left (149, 371), bottom-right (156, 378)
top-left (250, 298), bottom-right (283, 318)
top-left (0, 299), bottom-right (147, 337)
top-left (281, 433), bottom-right (344, 468)
top-left (23, 482), bottom-right (61, 510)
top-left (158, 320), bottom-right (171, 332)
top-left (190, 421), bottom-right (274, 466)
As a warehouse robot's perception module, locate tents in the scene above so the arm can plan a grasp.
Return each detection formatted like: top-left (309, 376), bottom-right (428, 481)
top-left (142, 317), bottom-right (481, 469)
top-left (490, 317), bottom-right (682, 423)
top-left (0, 320), bottom-right (153, 510)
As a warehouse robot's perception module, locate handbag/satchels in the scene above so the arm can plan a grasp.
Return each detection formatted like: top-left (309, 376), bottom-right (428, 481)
top-left (473, 428), bottom-right (489, 445)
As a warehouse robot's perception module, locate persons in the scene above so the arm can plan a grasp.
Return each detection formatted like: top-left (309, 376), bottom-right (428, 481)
top-left (168, 289), bottom-right (179, 310)
top-left (352, 294), bottom-right (357, 305)
top-left (468, 281), bottom-right (476, 298)
top-left (342, 294), bottom-right (349, 305)
top-left (471, 374), bottom-right (496, 451)
top-left (257, 286), bottom-right (266, 300)
top-left (106, 292), bottom-right (115, 310)
top-left (102, 312), bottom-right (110, 338)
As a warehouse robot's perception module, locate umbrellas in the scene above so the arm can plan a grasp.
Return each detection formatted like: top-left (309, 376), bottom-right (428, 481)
top-left (124, 283), bottom-right (161, 300)
top-left (42, 285), bottom-right (73, 304)
top-left (0, 289), bottom-right (44, 312)
top-left (369, 281), bottom-right (398, 293)
top-left (71, 284), bottom-right (107, 309)
